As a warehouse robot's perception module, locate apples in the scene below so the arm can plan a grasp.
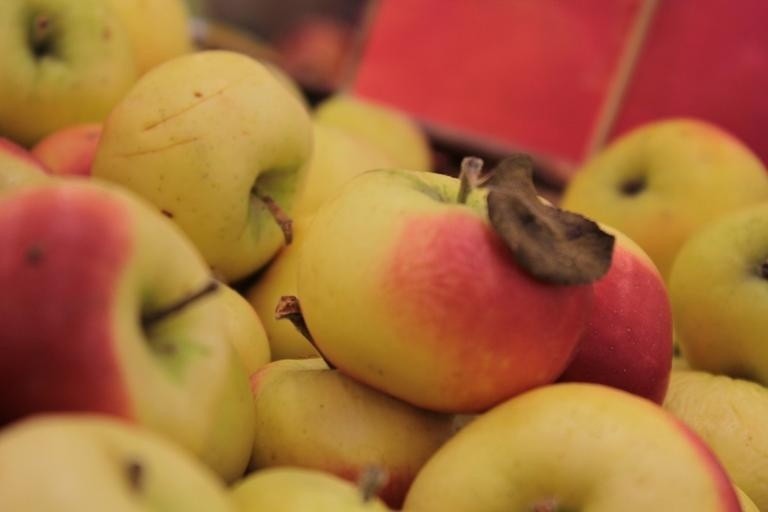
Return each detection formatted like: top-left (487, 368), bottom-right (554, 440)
top-left (0, 2), bottom-right (768, 512)
